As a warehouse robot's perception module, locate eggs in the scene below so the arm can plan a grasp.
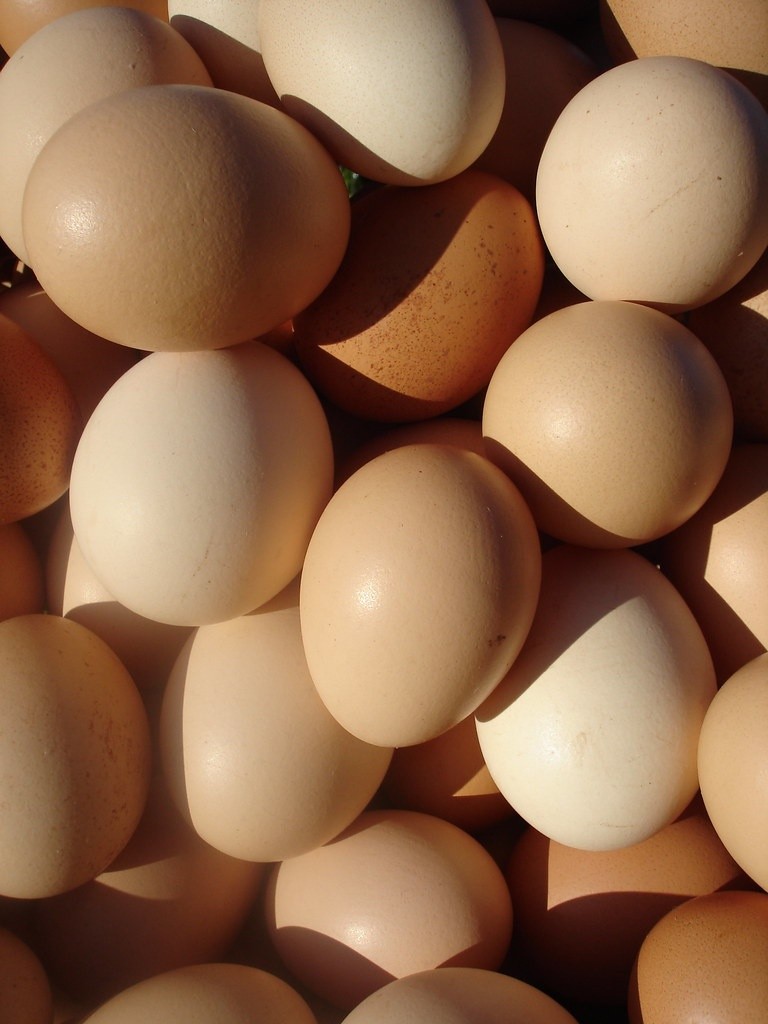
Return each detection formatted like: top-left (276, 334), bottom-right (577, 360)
top-left (0, 0), bottom-right (768, 1024)
